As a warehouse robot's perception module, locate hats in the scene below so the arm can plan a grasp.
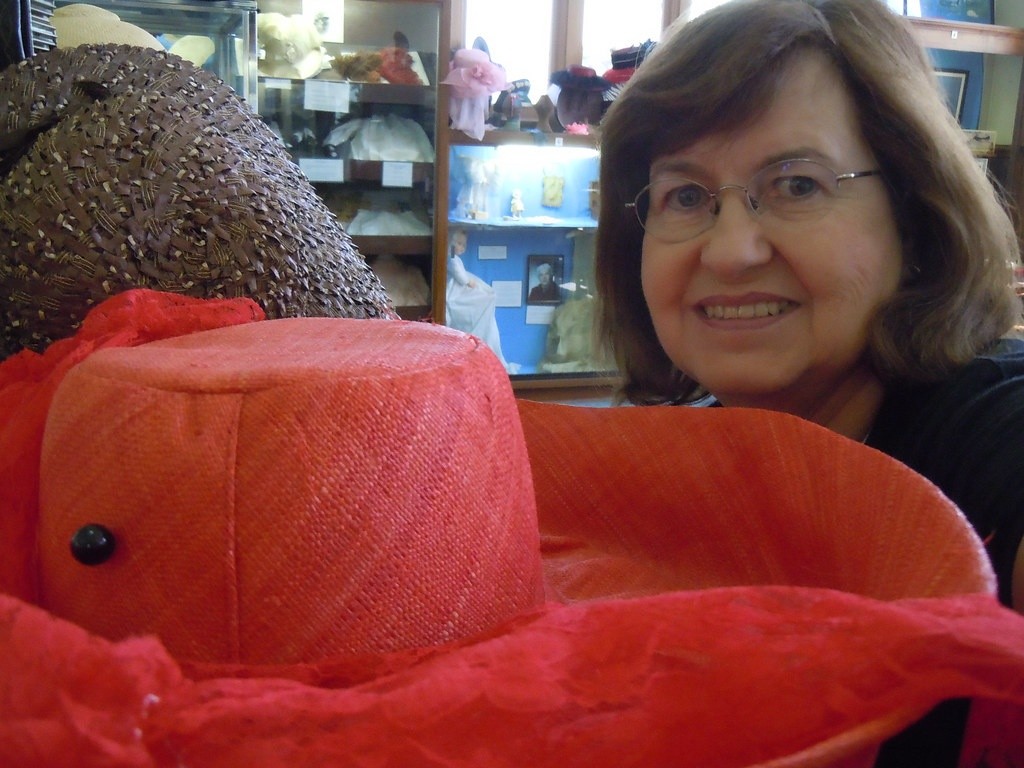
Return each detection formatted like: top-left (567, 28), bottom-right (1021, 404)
top-left (439, 48), bottom-right (507, 141)
top-left (0, 44), bottom-right (401, 370)
top-left (0, 290), bottom-right (1024, 768)
top-left (601, 40), bottom-right (657, 84)
top-left (550, 64), bottom-right (611, 92)
top-left (494, 79), bottom-right (529, 114)
top-left (49, 3), bottom-right (166, 54)
top-left (160, 34), bottom-right (216, 68)
top-left (239, 12), bottom-right (334, 78)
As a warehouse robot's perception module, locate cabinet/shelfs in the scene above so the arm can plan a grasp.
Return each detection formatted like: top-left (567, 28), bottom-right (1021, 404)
top-left (256, 0), bottom-right (452, 327)
top-left (441, 129), bottom-right (619, 391)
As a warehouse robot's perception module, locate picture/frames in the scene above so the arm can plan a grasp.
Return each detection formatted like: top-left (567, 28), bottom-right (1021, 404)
top-left (526, 254), bottom-right (564, 304)
top-left (934, 67), bottom-right (970, 123)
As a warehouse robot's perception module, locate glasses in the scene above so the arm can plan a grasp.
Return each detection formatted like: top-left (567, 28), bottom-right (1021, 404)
top-left (624, 159), bottom-right (882, 243)
top-left (287, 126), bottom-right (316, 149)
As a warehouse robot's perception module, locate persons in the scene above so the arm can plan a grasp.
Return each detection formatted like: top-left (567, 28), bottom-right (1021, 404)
top-left (596, 1), bottom-right (1024, 768)
top-left (446, 229), bottom-right (523, 374)
top-left (510, 189), bottom-right (525, 218)
top-left (528, 263), bottom-right (562, 300)
